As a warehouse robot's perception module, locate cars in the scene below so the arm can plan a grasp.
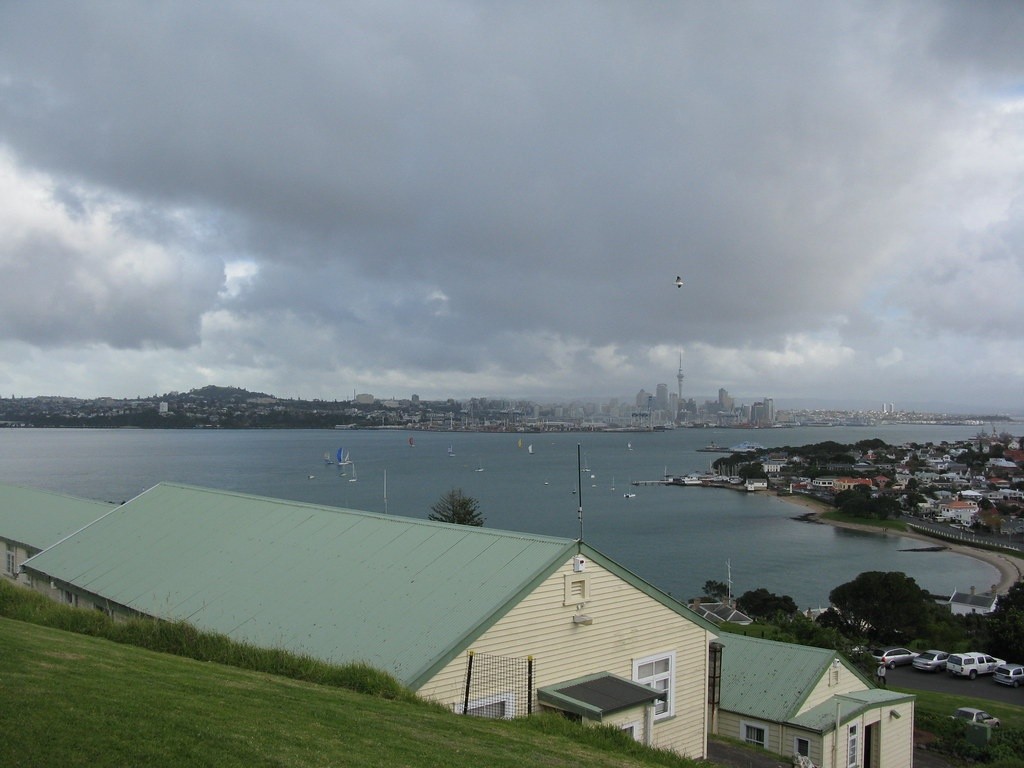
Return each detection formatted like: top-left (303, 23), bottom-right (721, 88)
top-left (994, 664), bottom-right (1024, 686)
top-left (913, 650), bottom-right (950, 670)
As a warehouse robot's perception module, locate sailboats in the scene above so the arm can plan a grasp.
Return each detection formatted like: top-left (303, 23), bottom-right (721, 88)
top-left (448, 444), bottom-right (456, 457)
top-left (582, 452), bottom-right (591, 473)
top-left (475, 460), bottom-right (484, 472)
top-left (623, 476), bottom-right (635, 499)
top-left (324, 448), bottom-right (351, 465)
top-left (611, 475), bottom-right (615, 492)
top-left (409, 436), bottom-right (415, 448)
top-left (628, 441), bottom-right (633, 450)
top-left (528, 445), bottom-right (534, 454)
top-left (518, 439), bottom-right (523, 448)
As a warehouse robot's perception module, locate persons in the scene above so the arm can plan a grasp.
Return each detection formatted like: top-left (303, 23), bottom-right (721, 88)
top-left (877, 663), bottom-right (886, 685)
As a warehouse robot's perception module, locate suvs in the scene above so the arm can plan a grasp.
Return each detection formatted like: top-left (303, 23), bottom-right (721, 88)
top-left (949, 707), bottom-right (1000, 730)
top-left (872, 645), bottom-right (922, 670)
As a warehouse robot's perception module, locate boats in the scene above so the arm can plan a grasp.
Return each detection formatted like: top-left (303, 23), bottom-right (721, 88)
top-left (339, 467), bottom-right (347, 477)
top-left (591, 475), bottom-right (596, 479)
top-left (592, 484), bottom-right (596, 488)
top-left (728, 443), bottom-right (763, 453)
top-left (309, 475), bottom-right (314, 479)
top-left (349, 464), bottom-right (358, 482)
top-left (695, 441), bottom-right (728, 452)
top-left (572, 489), bottom-right (577, 494)
top-left (544, 482), bottom-right (549, 485)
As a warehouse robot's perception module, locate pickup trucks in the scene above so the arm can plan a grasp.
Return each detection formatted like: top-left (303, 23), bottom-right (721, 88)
top-left (947, 653), bottom-right (1007, 680)
